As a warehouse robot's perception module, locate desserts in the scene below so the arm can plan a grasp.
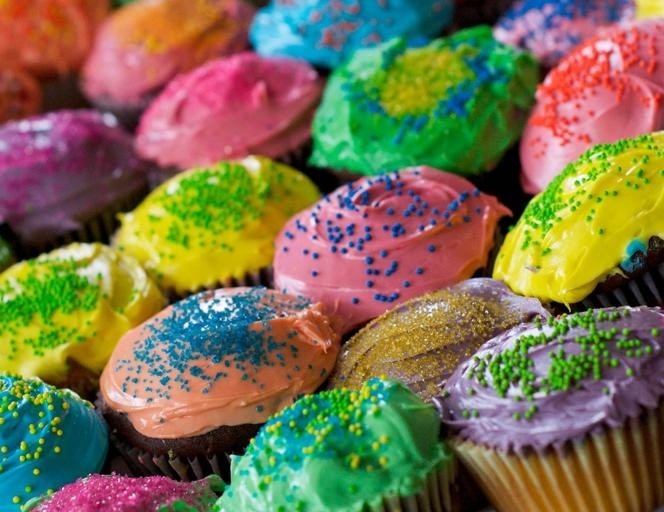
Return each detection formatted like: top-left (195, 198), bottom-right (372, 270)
top-left (1, 0), bottom-right (664, 511)
